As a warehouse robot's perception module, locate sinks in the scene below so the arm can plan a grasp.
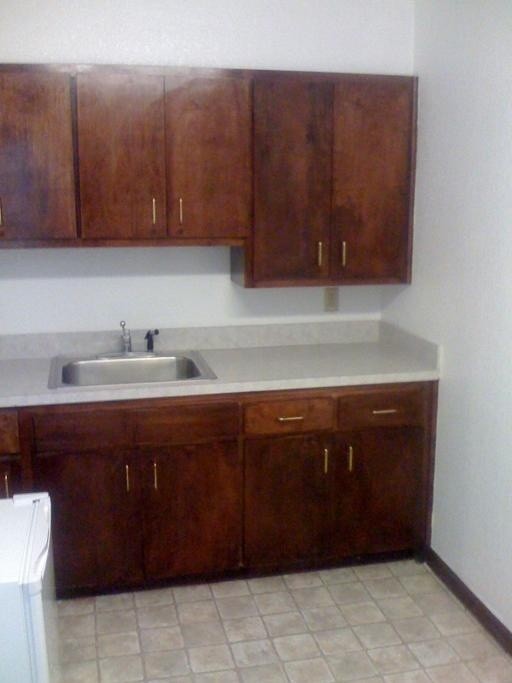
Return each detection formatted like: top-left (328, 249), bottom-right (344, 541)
top-left (45, 349), bottom-right (219, 391)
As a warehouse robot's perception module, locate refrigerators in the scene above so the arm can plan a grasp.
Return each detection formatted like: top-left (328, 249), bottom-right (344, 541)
top-left (0, 488), bottom-right (62, 682)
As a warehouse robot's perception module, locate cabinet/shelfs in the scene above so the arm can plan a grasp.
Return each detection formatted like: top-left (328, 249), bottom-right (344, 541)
top-left (242, 379), bottom-right (441, 580)
top-left (0, 407), bottom-right (31, 502)
top-left (74, 68), bottom-right (252, 252)
top-left (31, 392), bottom-right (243, 601)
top-left (230, 71), bottom-right (418, 286)
top-left (1, 64), bottom-right (77, 250)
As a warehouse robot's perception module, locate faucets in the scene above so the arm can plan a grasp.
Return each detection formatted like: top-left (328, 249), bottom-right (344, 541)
top-left (119, 320), bottom-right (132, 354)
